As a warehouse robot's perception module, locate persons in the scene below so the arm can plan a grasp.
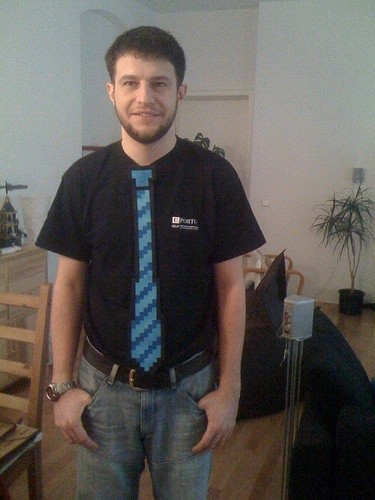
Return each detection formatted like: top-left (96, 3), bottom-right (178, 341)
top-left (33, 25), bottom-right (267, 497)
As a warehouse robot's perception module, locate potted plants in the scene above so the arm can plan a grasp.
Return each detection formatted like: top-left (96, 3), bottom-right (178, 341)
top-left (312, 180), bottom-right (375, 316)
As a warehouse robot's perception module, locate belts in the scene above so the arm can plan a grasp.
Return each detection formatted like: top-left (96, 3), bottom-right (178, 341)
top-left (82, 336), bottom-right (217, 392)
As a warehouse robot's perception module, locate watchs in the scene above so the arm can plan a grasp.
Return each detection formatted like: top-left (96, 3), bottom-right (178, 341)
top-left (45, 379), bottom-right (77, 402)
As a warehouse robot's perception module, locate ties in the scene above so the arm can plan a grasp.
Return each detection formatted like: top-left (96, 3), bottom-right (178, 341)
top-left (129, 168), bottom-right (165, 378)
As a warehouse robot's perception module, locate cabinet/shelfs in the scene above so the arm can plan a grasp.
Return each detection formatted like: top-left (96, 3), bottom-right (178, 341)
top-left (0, 245), bottom-right (49, 390)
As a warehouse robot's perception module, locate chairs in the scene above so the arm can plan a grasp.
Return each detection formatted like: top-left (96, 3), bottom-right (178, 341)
top-left (0, 283), bottom-right (53, 499)
